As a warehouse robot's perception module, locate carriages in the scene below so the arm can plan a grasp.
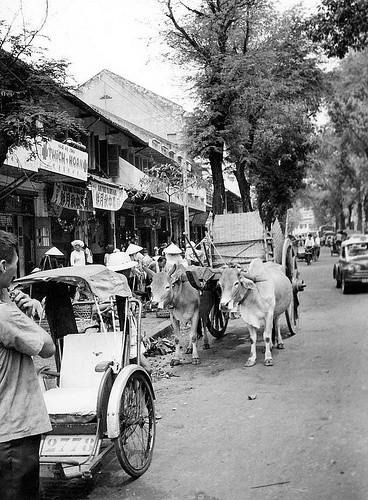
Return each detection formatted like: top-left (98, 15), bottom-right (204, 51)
top-left (140, 211), bottom-right (307, 367)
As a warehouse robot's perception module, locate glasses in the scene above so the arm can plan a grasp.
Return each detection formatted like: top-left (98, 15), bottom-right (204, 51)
top-left (75, 245), bottom-right (80, 247)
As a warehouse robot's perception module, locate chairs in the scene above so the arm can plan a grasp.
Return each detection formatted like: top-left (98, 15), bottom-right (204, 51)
top-left (37, 331), bottom-right (123, 423)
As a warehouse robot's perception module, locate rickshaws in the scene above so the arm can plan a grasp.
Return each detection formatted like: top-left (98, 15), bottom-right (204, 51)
top-left (1, 264), bottom-right (157, 488)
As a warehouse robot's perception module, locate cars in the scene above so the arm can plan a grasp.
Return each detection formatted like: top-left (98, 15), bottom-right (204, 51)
top-left (318, 225), bottom-right (368, 294)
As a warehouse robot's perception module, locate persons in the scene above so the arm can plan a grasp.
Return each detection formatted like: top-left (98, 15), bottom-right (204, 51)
top-left (0, 230), bottom-right (55, 500)
top-left (40, 237), bottom-right (205, 312)
top-left (294, 230), bottom-right (348, 261)
top-left (92, 252), bottom-right (162, 425)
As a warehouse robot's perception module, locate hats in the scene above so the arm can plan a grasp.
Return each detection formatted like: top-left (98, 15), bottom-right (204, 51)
top-left (186, 241), bottom-right (196, 247)
top-left (45, 246), bottom-right (64, 255)
top-left (126, 244), bottom-right (143, 255)
top-left (70, 240), bottom-right (84, 247)
top-left (162, 243), bottom-right (183, 254)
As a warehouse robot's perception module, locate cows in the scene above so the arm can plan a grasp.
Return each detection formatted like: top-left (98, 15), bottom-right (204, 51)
top-left (142, 263), bottom-right (209, 366)
top-left (209, 258), bottom-right (293, 367)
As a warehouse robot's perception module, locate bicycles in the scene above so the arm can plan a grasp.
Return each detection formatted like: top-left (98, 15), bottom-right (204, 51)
top-left (293, 239), bottom-right (320, 265)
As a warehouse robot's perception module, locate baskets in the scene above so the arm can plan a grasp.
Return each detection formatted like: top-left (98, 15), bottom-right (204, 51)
top-left (156, 310), bottom-right (170, 318)
